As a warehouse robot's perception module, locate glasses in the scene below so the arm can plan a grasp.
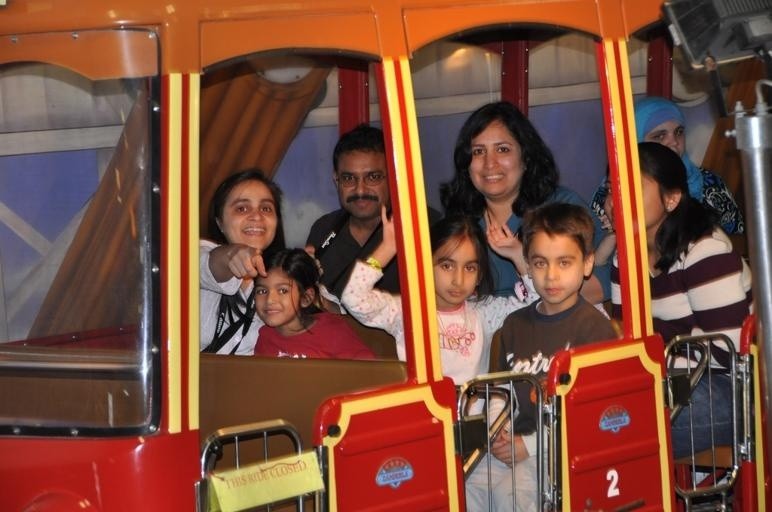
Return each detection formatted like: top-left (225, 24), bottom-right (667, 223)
top-left (336, 171), bottom-right (388, 186)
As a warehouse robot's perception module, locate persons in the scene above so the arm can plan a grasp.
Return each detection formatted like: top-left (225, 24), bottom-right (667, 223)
top-left (253, 246), bottom-right (373, 360)
top-left (197, 173), bottom-right (322, 354)
top-left (342, 203), bottom-right (536, 383)
top-left (468, 203), bottom-right (619, 510)
top-left (303, 123), bottom-right (440, 309)
top-left (439, 101), bottom-right (614, 306)
top-left (590, 95), bottom-right (748, 246)
top-left (603, 141), bottom-right (753, 495)
